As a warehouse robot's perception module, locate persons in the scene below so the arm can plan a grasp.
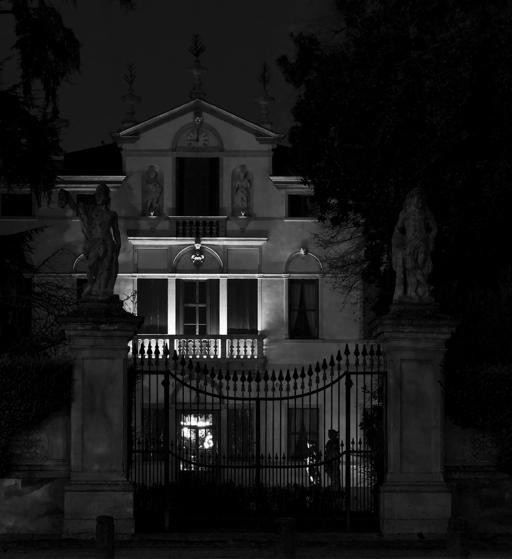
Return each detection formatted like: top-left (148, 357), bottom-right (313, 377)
top-left (322, 428), bottom-right (340, 489)
top-left (391, 185), bottom-right (440, 307)
top-left (231, 168), bottom-right (253, 219)
top-left (61, 180), bottom-right (124, 303)
top-left (140, 169), bottom-right (163, 217)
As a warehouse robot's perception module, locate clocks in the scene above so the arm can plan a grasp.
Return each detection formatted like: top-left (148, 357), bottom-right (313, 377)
top-left (187, 129), bottom-right (208, 152)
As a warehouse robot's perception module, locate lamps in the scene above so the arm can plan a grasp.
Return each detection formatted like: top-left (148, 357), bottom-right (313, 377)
top-left (191, 230), bottom-right (205, 267)
